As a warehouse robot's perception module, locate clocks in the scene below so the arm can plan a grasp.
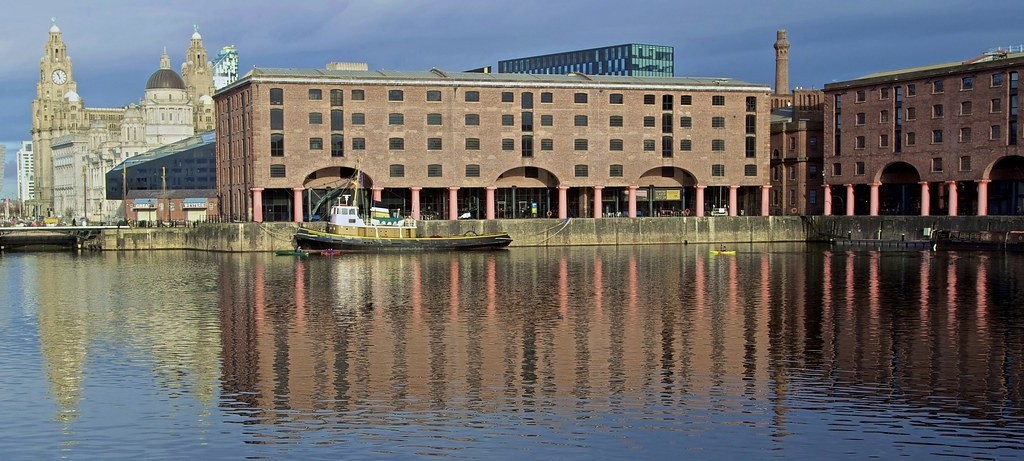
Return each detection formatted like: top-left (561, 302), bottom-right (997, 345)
top-left (52, 69), bottom-right (67, 85)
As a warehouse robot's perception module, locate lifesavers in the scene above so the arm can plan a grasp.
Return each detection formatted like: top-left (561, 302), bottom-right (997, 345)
top-left (684, 208), bottom-right (691, 216)
top-left (791, 207), bottom-right (799, 215)
top-left (546, 210), bottom-right (552, 217)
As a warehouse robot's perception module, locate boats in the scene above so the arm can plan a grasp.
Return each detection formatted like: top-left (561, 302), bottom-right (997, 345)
top-left (321, 251), bottom-right (341, 256)
top-left (274, 250), bottom-right (309, 258)
top-left (292, 156), bottom-right (514, 252)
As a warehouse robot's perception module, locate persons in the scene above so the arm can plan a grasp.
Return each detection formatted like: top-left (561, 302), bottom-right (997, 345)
top-left (720, 244), bottom-right (727, 251)
top-left (295, 246), bottom-right (302, 253)
top-left (325, 246), bottom-right (333, 252)
